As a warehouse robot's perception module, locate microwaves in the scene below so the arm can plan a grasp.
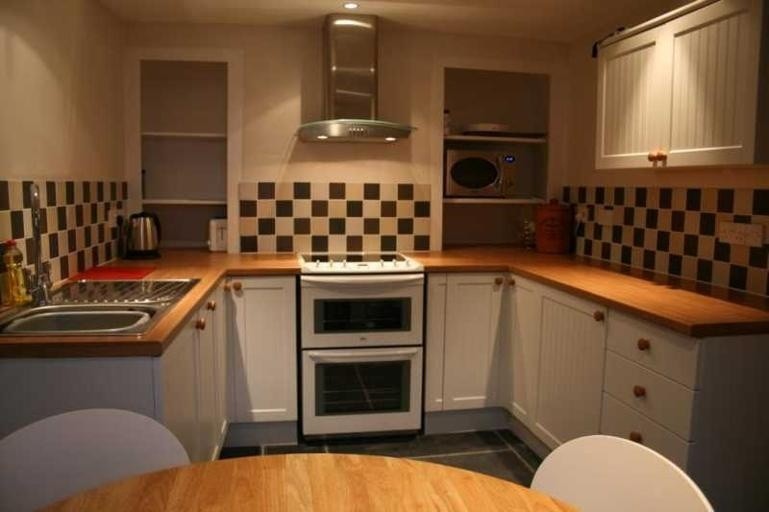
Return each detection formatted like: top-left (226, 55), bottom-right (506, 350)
top-left (447, 149), bottom-right (518, 197)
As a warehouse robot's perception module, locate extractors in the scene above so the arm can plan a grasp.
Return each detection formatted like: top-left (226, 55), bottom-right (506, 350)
top-left (293, 13), bottom-right (419, 144)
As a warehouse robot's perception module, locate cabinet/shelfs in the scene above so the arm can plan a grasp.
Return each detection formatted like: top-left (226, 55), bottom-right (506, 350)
top-left (603, 293), bottom-right (766, 508)
top-left (227, 271), bottom-right (300, 453)
top-left (538, 286), bottom-right (606, 467)
top-left (426, 271), bottom-right (507, 419)
top-left (155, 278), bottom-right (225, 470)
top-left (508, 270), bottom-right (533, 426)
top-left (126, 47), bottom-right (246, 251)
top-left (430, 59), bottom-right (555, 252)
top-left (593, 0), bottom-right (766, 173)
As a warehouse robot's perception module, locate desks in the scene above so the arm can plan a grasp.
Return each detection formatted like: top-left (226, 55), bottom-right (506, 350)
top-left (40, 449), bottom-right (589, 510)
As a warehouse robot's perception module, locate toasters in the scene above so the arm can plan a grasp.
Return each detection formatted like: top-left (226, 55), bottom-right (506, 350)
top-left (209, 218), bottom-right (228, 252)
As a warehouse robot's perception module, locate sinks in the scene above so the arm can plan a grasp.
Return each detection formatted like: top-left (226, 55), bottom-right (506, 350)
top-left (0, 302), bottom-right (174, 335)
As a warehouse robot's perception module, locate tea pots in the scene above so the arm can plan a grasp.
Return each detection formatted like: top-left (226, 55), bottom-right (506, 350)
top-left (126, 212), bottom-right (164, 260)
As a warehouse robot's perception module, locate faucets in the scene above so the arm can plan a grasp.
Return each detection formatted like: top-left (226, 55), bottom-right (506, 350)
top-left (30, 181), bottom-right (55, 307)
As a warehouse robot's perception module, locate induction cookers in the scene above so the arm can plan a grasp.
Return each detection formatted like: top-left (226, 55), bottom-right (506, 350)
top-left (297, 251), bottom-right (421, 283)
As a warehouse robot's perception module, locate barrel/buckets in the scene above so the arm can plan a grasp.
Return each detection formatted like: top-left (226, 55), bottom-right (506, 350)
top-left (533, 198), bottom-right (572, 255)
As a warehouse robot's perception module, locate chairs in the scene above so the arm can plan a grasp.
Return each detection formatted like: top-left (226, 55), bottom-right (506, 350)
top-left (529, 427), bottom-right (715, 509)
top-left (0, 406), bottom-right (192, 508)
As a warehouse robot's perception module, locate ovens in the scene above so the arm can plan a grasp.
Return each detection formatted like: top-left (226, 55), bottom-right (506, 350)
top-left (299, 276), bottom-right (424, 438)
